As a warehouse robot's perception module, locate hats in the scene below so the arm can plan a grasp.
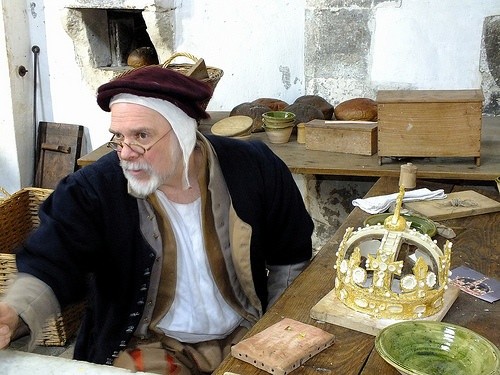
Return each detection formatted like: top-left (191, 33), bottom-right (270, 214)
top-left (97, 67), bottom-right (212, 120)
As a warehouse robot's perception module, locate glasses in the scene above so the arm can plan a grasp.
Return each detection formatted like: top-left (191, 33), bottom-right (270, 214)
top-left (107, 128), bottom-right (173, 156)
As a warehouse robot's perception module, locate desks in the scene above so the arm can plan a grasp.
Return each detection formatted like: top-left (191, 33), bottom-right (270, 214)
top-left (212, 176), bottom-right (500, 375)
top-left (76, 110), bottom-right (500, 182)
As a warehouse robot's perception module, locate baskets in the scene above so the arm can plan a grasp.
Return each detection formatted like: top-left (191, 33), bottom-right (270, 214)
top-left (109, 52), bottom-right (224, 127)
top-left (0, 186), bottom-right (88, 345)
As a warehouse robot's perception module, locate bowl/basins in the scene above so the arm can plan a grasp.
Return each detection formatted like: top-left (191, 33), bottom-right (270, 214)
top-left (374, 320), bottom-right (500, 375)
top-left (211, 115), bottom-right (254, 137)
top-left (262, 111), bottom-right (295, 144)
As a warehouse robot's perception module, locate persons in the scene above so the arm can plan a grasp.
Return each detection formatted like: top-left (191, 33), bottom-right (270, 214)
top-left (0, 65), bottom-right (315, 375)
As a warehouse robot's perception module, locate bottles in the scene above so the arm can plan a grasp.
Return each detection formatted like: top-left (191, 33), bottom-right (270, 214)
top-left (297, 122), bottom-right (305, 144)
top-left (399, 163), bottom-right (417, 190)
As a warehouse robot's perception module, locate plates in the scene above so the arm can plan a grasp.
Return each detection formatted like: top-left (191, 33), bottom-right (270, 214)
top-left (364, 212), bottom-right (437, 237)
